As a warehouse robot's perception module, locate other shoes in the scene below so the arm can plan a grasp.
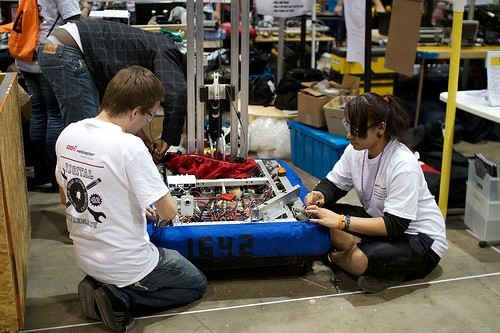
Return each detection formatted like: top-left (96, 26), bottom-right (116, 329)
top-left (93, 284), bottom-right (133, 333)
top-left (77, 274), bottom-right (102, 321)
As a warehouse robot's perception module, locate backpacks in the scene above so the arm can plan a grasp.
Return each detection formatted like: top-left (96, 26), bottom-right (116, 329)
top-left (248, 73), bottom-right (277, 106)
top-left (270, 44), bottom-right (296, 76)
top-left (249, 47), bottom-right (271, 75)
top-left (274, 68), bottom-right (324, 110)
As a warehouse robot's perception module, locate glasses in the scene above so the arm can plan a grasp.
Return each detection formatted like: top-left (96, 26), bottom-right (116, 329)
top-left (341, 117), bottom-right (376, 137)
top-left (142, 109), bottom-right (155, 122)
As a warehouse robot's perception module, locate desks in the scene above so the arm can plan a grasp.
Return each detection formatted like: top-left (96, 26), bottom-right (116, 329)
top-left (413, 46), bottom-right (500, 130)
top-left (254, 32), bottom-right (336, 55)
top-left (440, 90), bottom-right (500, 125)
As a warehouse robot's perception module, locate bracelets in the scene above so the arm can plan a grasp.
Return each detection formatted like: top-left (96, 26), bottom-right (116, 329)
top-left (337, 214), bottom-right (350, 232)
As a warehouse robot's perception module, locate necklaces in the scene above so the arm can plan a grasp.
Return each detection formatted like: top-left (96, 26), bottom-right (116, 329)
top-left (361, 135), bottom-right (391, 202)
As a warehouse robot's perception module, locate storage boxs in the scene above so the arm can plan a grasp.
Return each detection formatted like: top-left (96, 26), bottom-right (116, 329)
top-left (238, 51), bottom-right (397, 182)
top-left (464, 158), bottom-right (500, 242)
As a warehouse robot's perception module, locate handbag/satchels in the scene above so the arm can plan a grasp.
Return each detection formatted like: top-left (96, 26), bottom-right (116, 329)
top-left (8, 0), bottom-right (38, 63)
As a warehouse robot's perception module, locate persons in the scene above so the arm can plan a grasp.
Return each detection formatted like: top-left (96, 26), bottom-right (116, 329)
top-left (56, 66), bottom-right (206, 333)
top-left (304, 94), bottom-right (450, 294)
top-left (6, 0), bottom-right (279, 194)
top-left (326, 0), bottom-right (449, 47)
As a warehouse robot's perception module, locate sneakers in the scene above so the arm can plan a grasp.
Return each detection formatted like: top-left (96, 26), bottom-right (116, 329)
top-left (356, 272), bottom-right (405, 295)
